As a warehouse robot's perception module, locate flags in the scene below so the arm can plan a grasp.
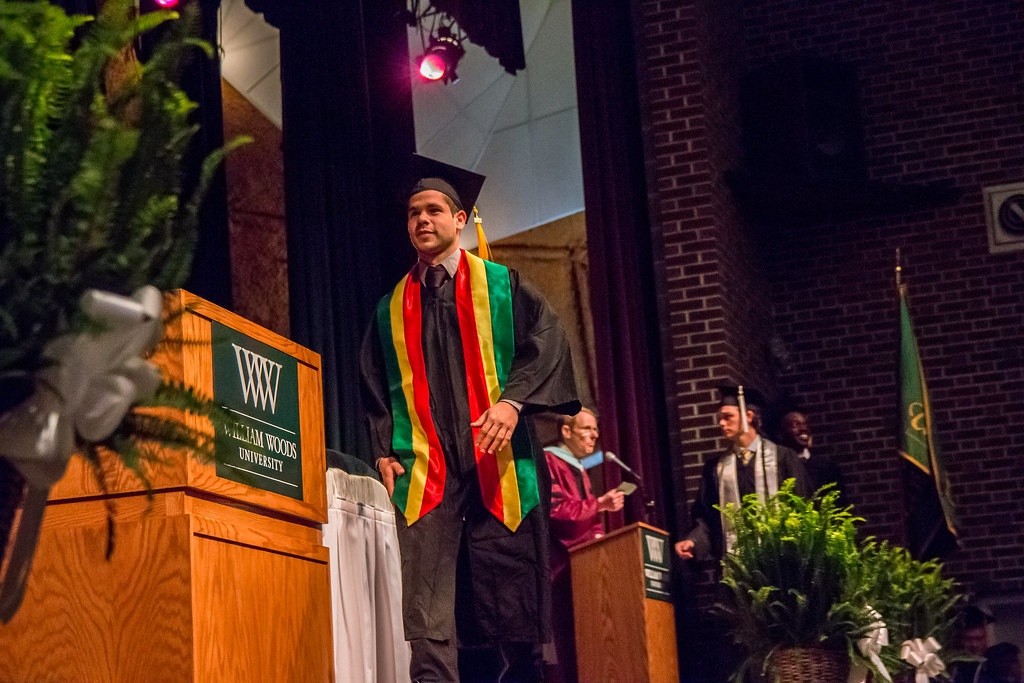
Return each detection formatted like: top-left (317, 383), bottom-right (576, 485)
top-left (898, 285), bottom-right (961, 563)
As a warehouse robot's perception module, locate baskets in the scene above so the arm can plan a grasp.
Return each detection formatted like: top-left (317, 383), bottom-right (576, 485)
top-left (749, 646), bottom-right (849, 683)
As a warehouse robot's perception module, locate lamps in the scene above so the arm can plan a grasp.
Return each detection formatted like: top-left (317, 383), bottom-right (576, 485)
top-left (413, 21), bottom-right (466, 86)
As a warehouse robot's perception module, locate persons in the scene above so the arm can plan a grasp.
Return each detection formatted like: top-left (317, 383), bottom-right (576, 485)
top-left (675, 386), bottom-right (840, 606)
top-left (349, 152), bottom-right (581, 683)
top-left (543, 408), bottom-right (626, 683)
top-left (945, 605), bottom-right (1024, 683)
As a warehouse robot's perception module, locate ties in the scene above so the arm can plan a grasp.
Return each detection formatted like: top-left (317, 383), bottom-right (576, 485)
top-left (426, 266), bottom-right (447, 288)
top-left (741, 450), bottom-right (754, 465)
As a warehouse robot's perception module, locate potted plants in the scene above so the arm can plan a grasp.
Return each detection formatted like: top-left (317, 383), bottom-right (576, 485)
top-left (711, 480), bottom-right (961, 683)
top-left (0, 0), bottom-right (256, 632)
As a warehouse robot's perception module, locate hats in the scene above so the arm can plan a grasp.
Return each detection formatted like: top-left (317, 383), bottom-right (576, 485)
top-left (394, 152), bottom-right (494, 262)
top-left (714, 384), bottom-right (770, 432)
